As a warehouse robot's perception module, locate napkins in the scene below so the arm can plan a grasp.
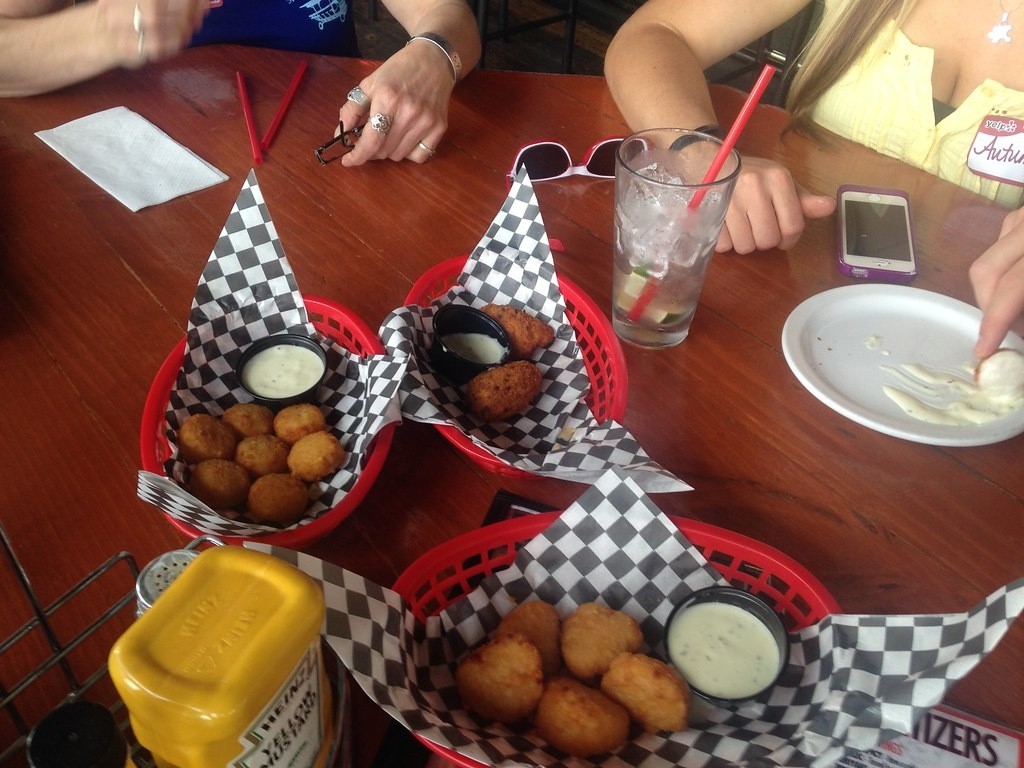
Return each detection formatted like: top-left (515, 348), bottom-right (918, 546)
top-left (33, 106), bottom-right (230, 213)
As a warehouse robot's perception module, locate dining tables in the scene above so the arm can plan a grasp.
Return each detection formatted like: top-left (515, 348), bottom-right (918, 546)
top-left (0, 42), bottom-right (1024, 768)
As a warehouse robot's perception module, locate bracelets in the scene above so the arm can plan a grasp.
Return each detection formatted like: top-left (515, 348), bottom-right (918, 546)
top-left (669, 125), bottom-right (729, 160)
top-left (407, 32), bottom-right (463, 81)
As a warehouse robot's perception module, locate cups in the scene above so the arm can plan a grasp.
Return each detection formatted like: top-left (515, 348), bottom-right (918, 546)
top-left (611, 129), bottom-right (740, 350)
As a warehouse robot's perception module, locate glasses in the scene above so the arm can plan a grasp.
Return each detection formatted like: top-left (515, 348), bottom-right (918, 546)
top-left (507, 136), bottom-right (656, 253)
top-left (314, 120), bottom-right (366, 167)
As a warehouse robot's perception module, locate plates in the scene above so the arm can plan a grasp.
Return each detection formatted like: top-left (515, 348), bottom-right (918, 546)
top-left (781, 284), bottom-right (1024, 446)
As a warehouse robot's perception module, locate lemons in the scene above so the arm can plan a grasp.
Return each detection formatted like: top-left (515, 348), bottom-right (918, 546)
top-left (617, 262), bottom-right (686, 325)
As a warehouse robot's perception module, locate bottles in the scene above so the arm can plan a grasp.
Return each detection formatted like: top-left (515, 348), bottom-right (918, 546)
top-left (133, 549), bottom-right (201, 618)
top-left (27, 702), bottom-right (136, 768)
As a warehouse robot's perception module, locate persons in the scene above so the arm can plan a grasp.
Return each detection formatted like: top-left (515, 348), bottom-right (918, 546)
top-left (0, 0), bottom-right (483, 167)
top-left (601, 0), bottom-right (1024, 361)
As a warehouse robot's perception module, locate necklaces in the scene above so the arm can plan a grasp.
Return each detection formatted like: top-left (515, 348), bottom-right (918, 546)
top-left (988, 0), bottom-right (1022, 44)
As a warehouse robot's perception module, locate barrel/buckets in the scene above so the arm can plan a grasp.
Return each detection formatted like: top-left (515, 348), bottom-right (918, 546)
top-left (109, 545), bottom-right (335, 768)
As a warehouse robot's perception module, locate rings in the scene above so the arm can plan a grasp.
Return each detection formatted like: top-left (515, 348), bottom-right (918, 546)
top-left (133, 5), bottom-right (141, 33)
top-left (419, 142), bottom-right (435, 156)
top-left (140, 32), bottom-right (143, 51)
top-left (367, 112), bottom-right (391, 135)
top-left (346, 85), bottom-right (370, 109)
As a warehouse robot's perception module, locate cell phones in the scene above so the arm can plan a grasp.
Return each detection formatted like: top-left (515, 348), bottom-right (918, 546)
top-left (835, 184), bottom-right (918, 282)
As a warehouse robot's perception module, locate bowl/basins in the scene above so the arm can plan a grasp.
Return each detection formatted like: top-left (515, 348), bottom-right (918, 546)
top-left (663, 587), bottom-right (788, 706)
top-left (430, 302), bottom-right (511, 385)
top-left (237, 334), bottom-right (328, 412)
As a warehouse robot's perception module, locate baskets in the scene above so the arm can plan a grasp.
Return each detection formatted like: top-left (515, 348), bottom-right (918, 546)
top-left (386, 510), bottom-right (842, 768)
top-left (400, 254), bottom-right (627, 505)
top-left (140, 292), bottom-right (396, 547)
top-left (0, 537), bottom-right (348, 768)
